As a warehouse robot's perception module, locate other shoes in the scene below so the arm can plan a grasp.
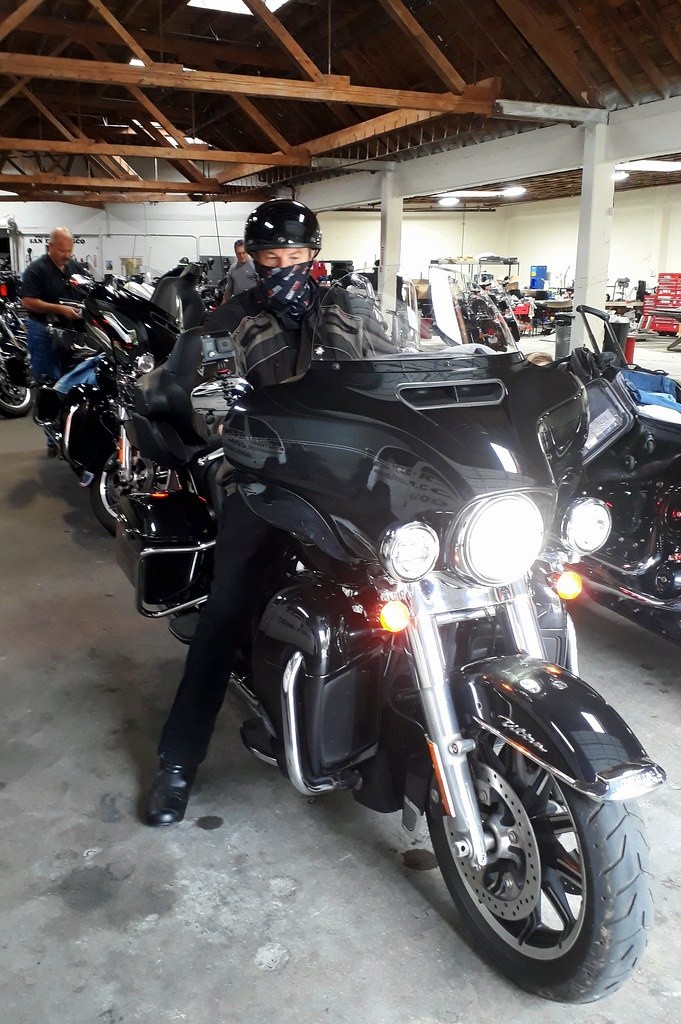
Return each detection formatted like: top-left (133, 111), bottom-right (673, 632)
top-left (48, 445), bottom-right (59, 459)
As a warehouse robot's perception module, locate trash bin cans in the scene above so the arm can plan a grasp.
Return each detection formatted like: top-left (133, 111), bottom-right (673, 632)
top-left (603, 314), bottom-right (630, 356)
top-left (554, 312), bottom-right (573, 362)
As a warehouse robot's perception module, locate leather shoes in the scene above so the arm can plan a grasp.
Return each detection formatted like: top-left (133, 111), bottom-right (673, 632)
top-left (147, 751), bottom-right (199, 825)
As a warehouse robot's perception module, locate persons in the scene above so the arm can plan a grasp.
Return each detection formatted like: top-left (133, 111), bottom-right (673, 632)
top-left (21, 226), bottom-right (90, 460)
top-left (220, 239), bottom-right (257, 306)
top-left (145, 198), bottom-right (400, 827)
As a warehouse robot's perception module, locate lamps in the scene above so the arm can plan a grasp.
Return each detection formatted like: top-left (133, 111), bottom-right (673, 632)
top-left (428, 159), bottom-right (681, 206)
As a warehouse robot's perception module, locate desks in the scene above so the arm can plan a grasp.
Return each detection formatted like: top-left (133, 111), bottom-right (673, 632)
top-left (605, 301), bottom-right (643, 313)
top-left (545, 299), bottom-right (573, 313)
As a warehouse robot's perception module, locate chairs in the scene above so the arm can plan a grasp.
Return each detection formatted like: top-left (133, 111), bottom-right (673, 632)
top-left (131, 321), bottom-right (228, 474)
top-left (349, 293), bottom-right (401, 357)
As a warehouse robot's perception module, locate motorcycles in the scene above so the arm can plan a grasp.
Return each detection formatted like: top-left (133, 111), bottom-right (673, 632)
top-left (122, 264), bottom-right (669, 1006)
top-left (1, 268), bottom-right (680, 639)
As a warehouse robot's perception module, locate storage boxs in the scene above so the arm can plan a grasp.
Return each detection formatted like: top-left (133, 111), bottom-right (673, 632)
top-left (412, 278), bottom-right (430, 300)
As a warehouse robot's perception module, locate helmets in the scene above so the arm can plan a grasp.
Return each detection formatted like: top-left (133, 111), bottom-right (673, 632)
top-left (242, 197), bottom-right (322, 252)
